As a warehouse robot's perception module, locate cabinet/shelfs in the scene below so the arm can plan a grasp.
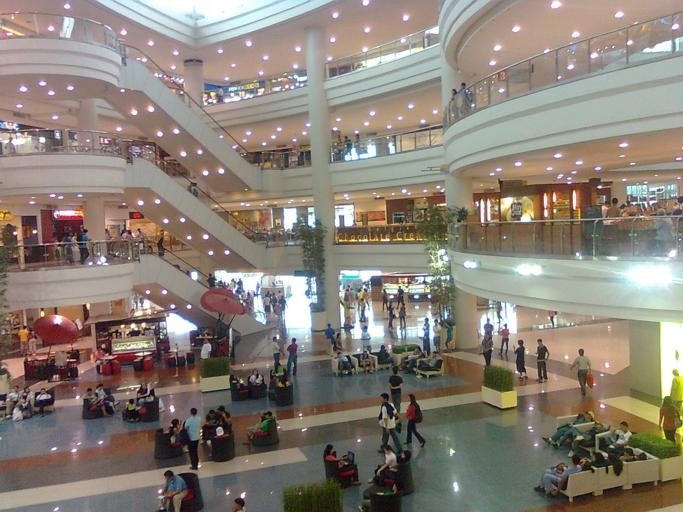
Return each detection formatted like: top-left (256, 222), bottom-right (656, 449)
top-left (111, 335), bottom-right (157, 365)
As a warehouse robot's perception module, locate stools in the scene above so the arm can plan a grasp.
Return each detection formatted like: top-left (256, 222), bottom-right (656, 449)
top-left (187, 352), bottom-right (194, 364)
top-left (95, 360), bottom-right (122, 376)
top-left (177, 356), bottom-right (185, 366)
top-left (168, 356), bottom-right (176, 368)
top-left (133, 357), bottom-right (154, 372)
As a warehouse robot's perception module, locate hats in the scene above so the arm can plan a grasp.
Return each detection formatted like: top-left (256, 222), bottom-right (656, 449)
top-left (588, 410), bottom-right (594, 418)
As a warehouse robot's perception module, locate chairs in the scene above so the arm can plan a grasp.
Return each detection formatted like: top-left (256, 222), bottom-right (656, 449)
top-left (139, 397), bottom-right (159, 422)
top-left (35, 391), bottom-right (54, 414)
top-left (83, 393), bottom-right (102, 419)
top-left (168, 473), bottom-right (203, 512)
top-left (325, 451), bottom-right (416, 512)
top-left (96, 388), bottom-right (111, 395)
top-left (551, 415), bottom-right (660, 504)
top-left (231, 369), bottom-right (294, 407)
top-left (155, 423), bottom-right (235, 462)
top-left (331, 350), bottom-right (444, 379)
top-left (253, 415), bottom-right (279, 447)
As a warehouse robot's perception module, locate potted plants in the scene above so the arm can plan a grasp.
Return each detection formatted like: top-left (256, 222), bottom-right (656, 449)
top-left (481, 363), bottom-right (518, 409)
top-left (627, 432), bottom-right (683, 482)
top-left (199, 356), bottom-right (231, 393)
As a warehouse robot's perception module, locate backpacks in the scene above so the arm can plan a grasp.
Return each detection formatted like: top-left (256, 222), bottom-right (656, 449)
top-left (414, 402), bottom-right (423, 422)
top-left (180, 429), bottom-right (190, 446)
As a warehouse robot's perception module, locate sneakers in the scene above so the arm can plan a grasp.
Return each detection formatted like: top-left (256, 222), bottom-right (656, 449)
top-left (354, 480), bottom-right (361, 485)
top-left (404, 440), bottom-right (412, 444)
top-left (534, 485), bottom-right (545, 492)
top-left (542, 437), bottom-right (574, 457)
top-left (190, 465), bottom-right (198, 469)
top-left (368, 478), bottom-right (375, 483)
top-left (518, 375), bottom-right (548, 384)
top-left (420, 440), bottom-right (425, 447)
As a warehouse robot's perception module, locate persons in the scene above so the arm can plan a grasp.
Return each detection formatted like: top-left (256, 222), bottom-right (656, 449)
top-left (324, 444), bottom-right (411, 511)
top-left (232, 497), bottom-right (245, 511)
top-left (344, 135), bottom-right (352, 159)
top-left (459, 82), bottom-right (473, 115)
top-left (2, 327), bottom-right (155, 423)
top-left (203, 92), bottom-right (213, 105)
top-left (481, 300), bottom-right (591, 396)
top-left (4, 137), bottom-right (16, 154)
top-left (202, 337), bottom-right (298, 399)
top-left (188, 176), bottom-right (198, 197)
top-left (451, 88), bottom-right (459, 119)
top-left (169, 405), bottom-right (272, 470)
top-left (534, 369), bottom-right (682, 497)
top-left (326, 286), bottom-right (452, 373)
top-left (51, 227), bottom-right (165, 265)
top-left (159, 469), bottom-right (189, 511)
top-left (209, 273), bottom-right (286, 318)
top-left (601, 195), bottom-right (683, 257)
top-left (354, 134), bottom-right (361, 159)
top-left (377, 366), bottom-right (426, 453)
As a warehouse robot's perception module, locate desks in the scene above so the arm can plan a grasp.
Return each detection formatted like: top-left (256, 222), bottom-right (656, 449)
top-left (133, 352), bottom-right (153, 359)
top-left (99, 355), bottom-right (117, 361)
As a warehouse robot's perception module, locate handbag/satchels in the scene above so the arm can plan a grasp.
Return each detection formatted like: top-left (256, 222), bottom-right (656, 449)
top-left (586, 374), bottom-right (595, 389)
